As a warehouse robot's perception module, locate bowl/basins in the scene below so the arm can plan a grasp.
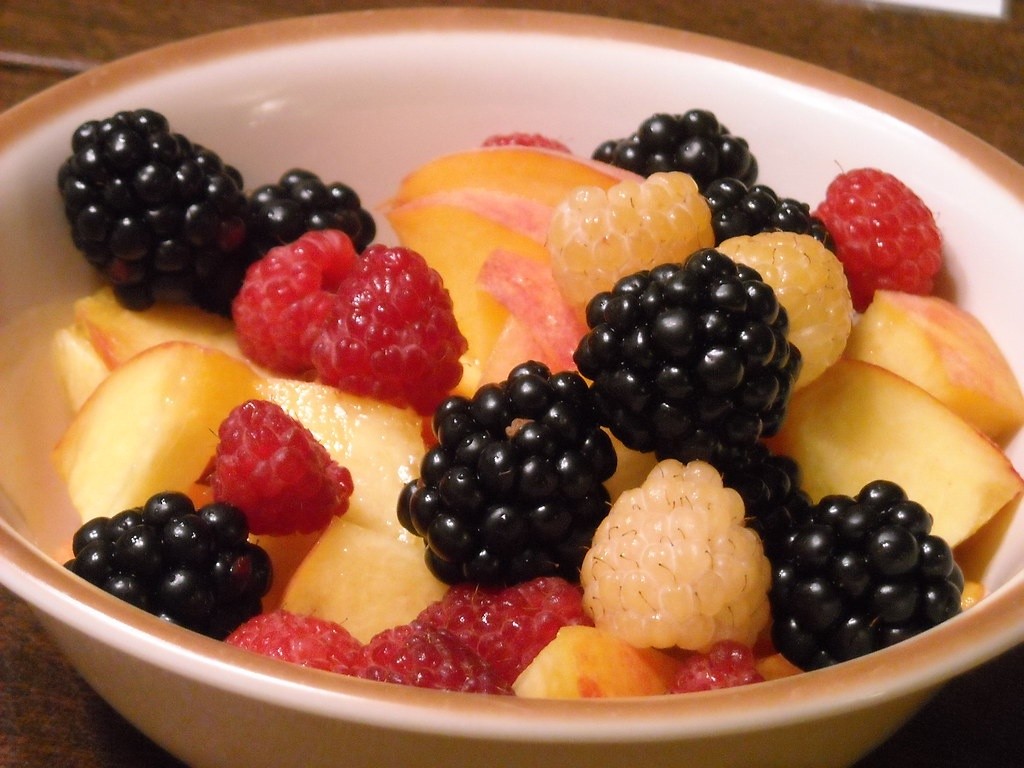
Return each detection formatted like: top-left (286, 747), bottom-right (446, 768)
top-left (0, 5), bottom-right (1024, 768)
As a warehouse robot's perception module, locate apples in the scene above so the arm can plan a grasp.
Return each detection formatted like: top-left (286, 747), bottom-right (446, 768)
top-left (53, 145), bottom-right (1024, 699)
